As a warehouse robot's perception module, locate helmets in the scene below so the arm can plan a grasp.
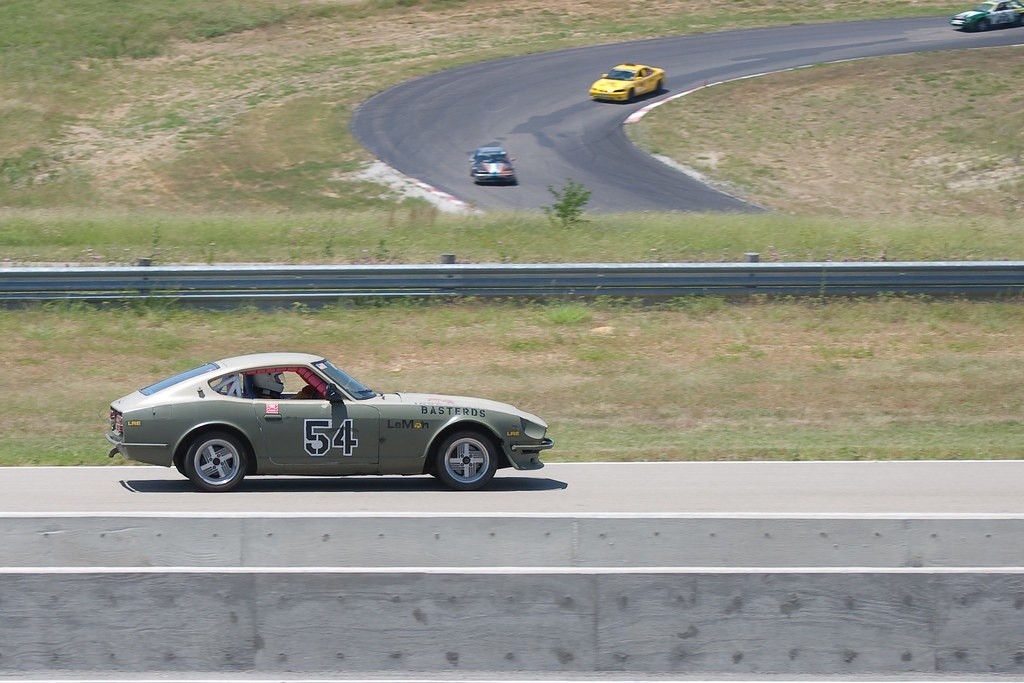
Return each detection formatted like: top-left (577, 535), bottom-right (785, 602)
top-left (252, 372), bottom-right (284, 393)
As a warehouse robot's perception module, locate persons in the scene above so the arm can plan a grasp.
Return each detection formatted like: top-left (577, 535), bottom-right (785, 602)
top-left (251, 367), bottom-right (322, 403)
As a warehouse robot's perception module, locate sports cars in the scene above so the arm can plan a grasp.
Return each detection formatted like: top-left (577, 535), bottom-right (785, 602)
top-left (106, 351), bottom-right (554, 491)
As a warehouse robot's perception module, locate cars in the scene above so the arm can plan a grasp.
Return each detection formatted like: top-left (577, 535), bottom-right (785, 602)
top-left (470, 147), bottom-right (517, 185)
top-left (949, 0), bottom-right (1024, 32)
top-left (588, 63), bottom-right (666, 103)
